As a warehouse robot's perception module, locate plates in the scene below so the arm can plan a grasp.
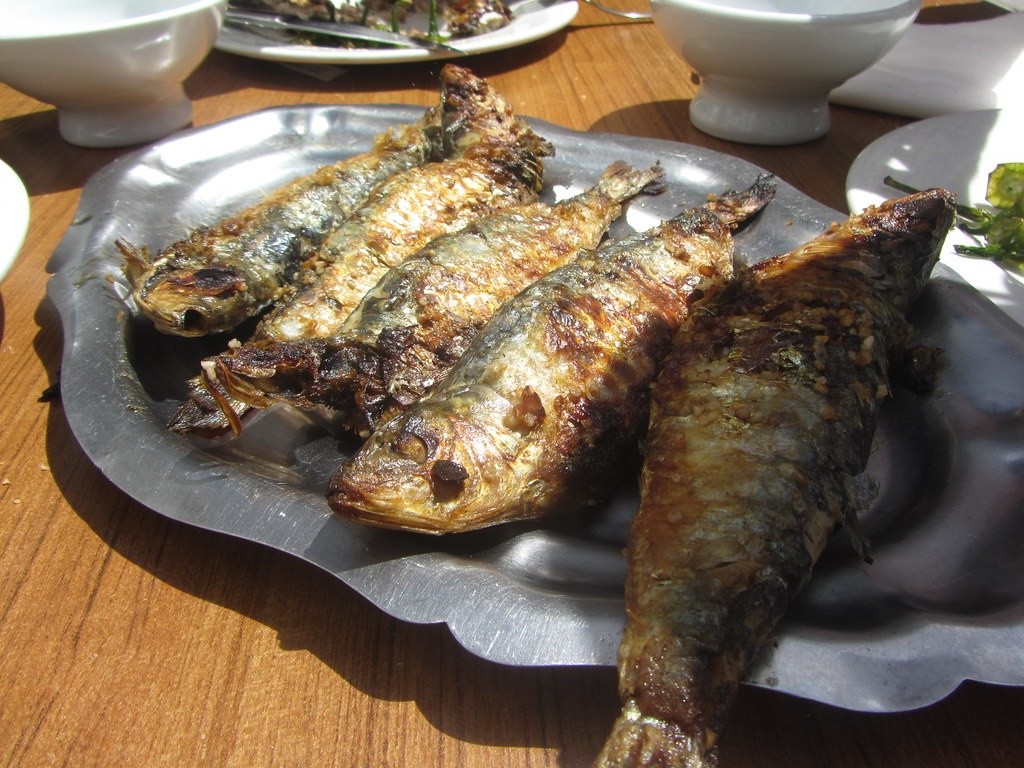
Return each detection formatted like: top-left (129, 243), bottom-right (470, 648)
top-left (40, 106), bottom-right (1024, 710)
top-left (210, 1), bottom-right (579, 64)
top-left (848, 107), bottom-right (1024, 349)
top-left (0, 157), bottom-right (30, 282)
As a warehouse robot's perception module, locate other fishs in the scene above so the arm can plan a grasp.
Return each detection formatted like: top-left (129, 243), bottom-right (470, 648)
top-left (113, 63), bottom-right (958, 768)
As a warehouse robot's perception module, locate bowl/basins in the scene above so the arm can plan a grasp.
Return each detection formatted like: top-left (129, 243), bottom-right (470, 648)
top-left (0, 0), bottom-right (224, 150)
top-left (646, 0), bottom-right (921, 147)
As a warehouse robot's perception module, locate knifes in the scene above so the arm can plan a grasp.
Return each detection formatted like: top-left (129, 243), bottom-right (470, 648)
top-left (213, 5), bottom-right (426, 49)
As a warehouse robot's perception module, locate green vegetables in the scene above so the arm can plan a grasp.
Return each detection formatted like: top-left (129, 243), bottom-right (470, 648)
top-left (303, 0), bottom-right (447, 48)
top-left (882, 162), bottom-right (1024, 266)
